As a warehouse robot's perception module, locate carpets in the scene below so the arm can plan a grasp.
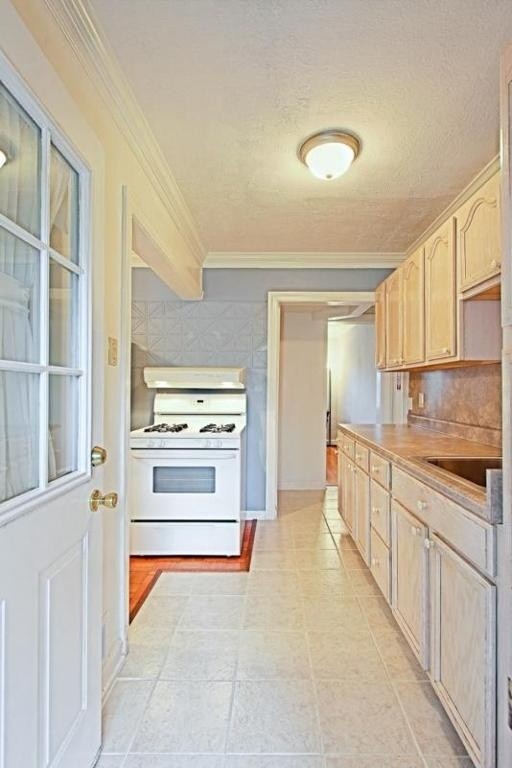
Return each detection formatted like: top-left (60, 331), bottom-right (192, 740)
top-left (127, 520), bottom-right (258, 623)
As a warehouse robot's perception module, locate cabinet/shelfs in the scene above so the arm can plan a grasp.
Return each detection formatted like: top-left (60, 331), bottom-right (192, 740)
top-left (344, 434), bottom-right (371, 561)
top-left (369, 453), bottom-right (392, 604)
top-left (338, 432), bottom-right (350, 520)
top-left (376, 156), bottom-right (501, 372)
top-left (389, 462), bottom-right (493, 767)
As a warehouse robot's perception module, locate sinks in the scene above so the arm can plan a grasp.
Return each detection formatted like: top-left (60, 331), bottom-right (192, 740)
top-left (412, 454), bottom-right (502, 494)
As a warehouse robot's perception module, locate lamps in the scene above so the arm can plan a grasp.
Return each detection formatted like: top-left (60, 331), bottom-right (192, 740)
top-left (297, 129), bottom-right (362, 180)
top-left (0, 143), bottom-right (10, 169)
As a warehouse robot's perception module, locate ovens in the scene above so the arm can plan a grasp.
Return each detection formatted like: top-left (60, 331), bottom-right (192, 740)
top-left (129, 447), bottom-right (240, 558)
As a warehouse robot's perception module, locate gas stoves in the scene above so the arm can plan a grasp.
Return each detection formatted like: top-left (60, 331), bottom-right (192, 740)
top-left (129, 414), bottom-right (247, 450)
